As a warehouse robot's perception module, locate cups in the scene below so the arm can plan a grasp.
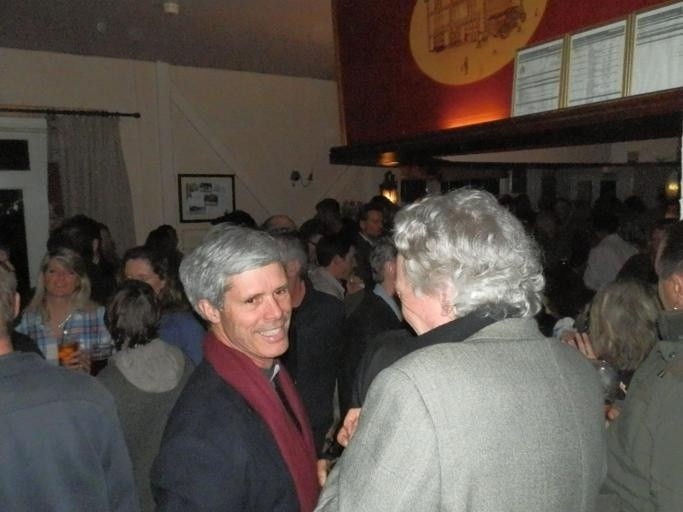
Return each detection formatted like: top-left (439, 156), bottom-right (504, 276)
top-left (55, 333), bottom-right (78, 367)
top-left (592, 359), bottom-right (621, 403)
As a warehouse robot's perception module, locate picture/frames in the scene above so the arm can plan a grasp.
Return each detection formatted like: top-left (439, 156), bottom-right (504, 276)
top-left (176, 170), bottom-right (236, 224)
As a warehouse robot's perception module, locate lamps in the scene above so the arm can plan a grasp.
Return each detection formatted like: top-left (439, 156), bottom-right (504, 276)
top-left (376, 169), bottom-right (399, 205)
